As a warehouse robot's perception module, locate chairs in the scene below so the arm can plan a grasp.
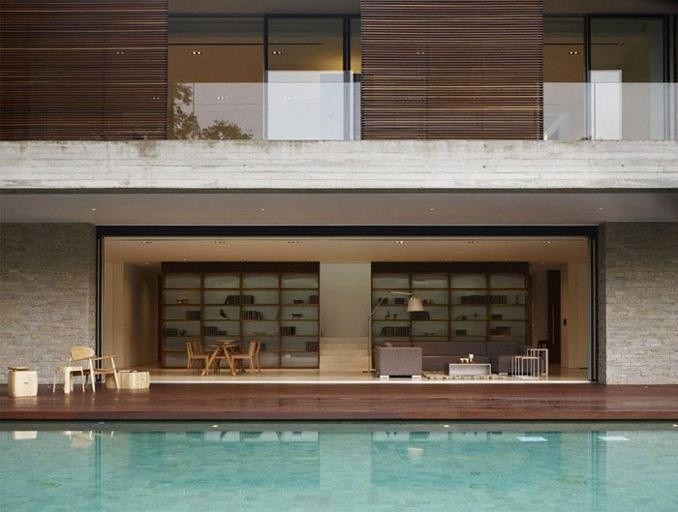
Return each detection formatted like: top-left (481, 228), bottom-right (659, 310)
top-left (183, 341), bottom-right (211, 376)
top-left (230, 341), bottom-right (261, 376)
top-left (66, 345), bottom-right (121, 393)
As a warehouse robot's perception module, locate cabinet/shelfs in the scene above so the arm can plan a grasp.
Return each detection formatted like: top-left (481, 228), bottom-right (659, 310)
top-left (160, 269), bottom-right (319, 354)
top-left (371, 265), bottom-right (529, 340)
top-left (511, 347), bottom-right (552, 378)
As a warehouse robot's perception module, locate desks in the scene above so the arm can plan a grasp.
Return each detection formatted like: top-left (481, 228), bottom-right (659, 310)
top-left (200, 342), bottom-right (241, 376)
top-left (444, 361), bottom-right (492, 376)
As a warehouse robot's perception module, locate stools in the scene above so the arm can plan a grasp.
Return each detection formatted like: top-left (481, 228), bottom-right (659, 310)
top-left (52, 365), bottom-right (86, 395)
top-left (6, 365), bottom-right (39, 398)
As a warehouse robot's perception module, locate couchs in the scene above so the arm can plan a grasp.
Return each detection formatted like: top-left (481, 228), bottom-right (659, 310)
top-left (373, 338), bottom-right (533, 378)
